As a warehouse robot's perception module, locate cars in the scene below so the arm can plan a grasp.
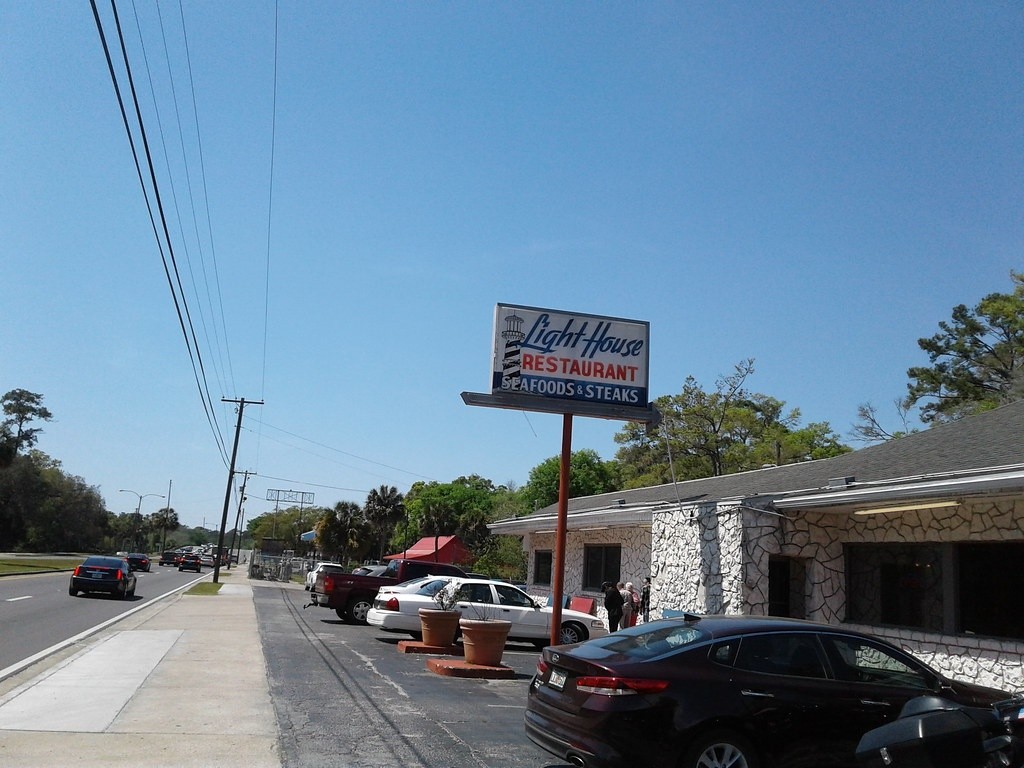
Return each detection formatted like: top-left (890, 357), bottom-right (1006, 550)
top-left (178, 542), bottom-right (233, 568)
top-left (158, 551), bottom-right (181, 567)
top-left (351, 565), bottom-right (387, 578)
top-left (305, 562), bottom-right (344, 591)
top-left (855, 688), bottom-right (1024, 767)
top-left (378, 573), bottom-right (453, 599)
top-left (366, 576), bottom-right (609, 651)
top-left (179, 554), bottom-right (201, 572)
top-left (125, 553), bottom-right (152, 572)
top-left (67, 556), bottom-right (136, 600)
top-left (523, 612), bottom-right (1015, 767)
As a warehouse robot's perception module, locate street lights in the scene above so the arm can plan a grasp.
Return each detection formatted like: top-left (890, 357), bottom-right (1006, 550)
top-left (119, 489), bottom-right (166, 515)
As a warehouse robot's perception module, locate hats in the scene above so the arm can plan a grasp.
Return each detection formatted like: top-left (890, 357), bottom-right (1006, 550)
top-left (601, 581), bottom-right (612, 591)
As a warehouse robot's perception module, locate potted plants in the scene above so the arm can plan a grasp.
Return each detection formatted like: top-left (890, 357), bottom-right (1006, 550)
top-left (457, 603), bottom-right (512, 667)
top-left (417, 580), bottom-right (465, 646)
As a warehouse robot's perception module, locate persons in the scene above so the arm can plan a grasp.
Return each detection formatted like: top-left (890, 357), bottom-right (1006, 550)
top-left (626, 582), bottom-right (641, 628)
top-left (601, 581), bottom-right (625, 634)
top-left (641, 576), bottom-right (652, 622)
top-left (616, 581), bottom-right (633, 631)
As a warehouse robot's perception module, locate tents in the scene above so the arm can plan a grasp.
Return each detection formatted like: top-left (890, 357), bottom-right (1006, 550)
top-left (384, 535), bottom-right (469, 566)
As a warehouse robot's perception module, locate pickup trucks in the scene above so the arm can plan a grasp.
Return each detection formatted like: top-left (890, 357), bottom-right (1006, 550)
top-left (309, 558), bottom-right (475, 623)
